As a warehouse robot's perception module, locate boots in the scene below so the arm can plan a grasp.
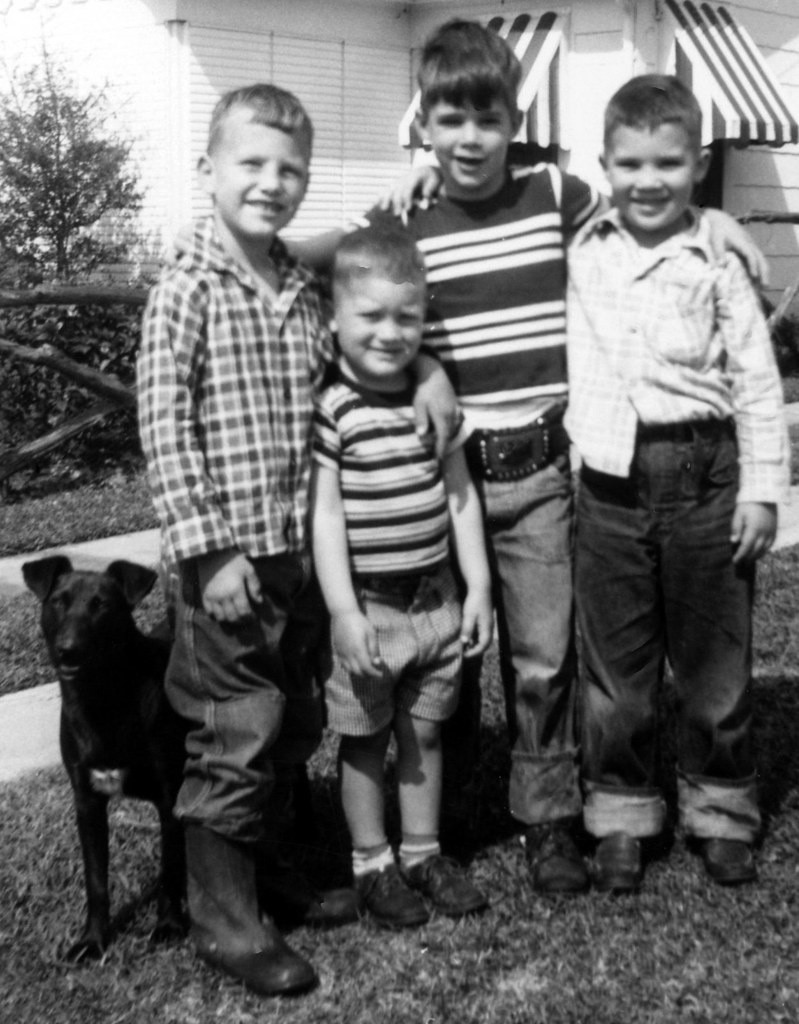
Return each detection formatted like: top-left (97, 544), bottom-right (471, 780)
top-left (185, 828), bottom-right (313, 993)
top-left (255, 823), bottom-right (361, 928)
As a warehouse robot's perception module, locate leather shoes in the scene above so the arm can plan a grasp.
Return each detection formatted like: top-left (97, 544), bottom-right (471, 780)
top-left (405, 853), bottom-right (487, 912)
top-left (592, 830), bottom-right (642, 892)
top-left (354, 862), bottom-right (430, 926)
top-left (704, 836), bottom-right (759, 881)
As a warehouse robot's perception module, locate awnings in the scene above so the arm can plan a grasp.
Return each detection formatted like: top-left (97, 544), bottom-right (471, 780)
top-left (397, 8), bottom-right (570, 153)
top-left (654, 0), bottom-right (799, 149)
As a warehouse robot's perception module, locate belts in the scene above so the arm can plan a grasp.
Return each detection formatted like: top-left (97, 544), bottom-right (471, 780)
top-left (636, 417), bottom-right (724, 443)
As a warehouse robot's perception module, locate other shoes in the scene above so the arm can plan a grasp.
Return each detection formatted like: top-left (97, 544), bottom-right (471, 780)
top-left (529, 818), bottom-right (592, 894)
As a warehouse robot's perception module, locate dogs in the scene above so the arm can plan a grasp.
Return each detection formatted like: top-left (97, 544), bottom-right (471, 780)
top-left (18, 554), bottom-right (200, 973)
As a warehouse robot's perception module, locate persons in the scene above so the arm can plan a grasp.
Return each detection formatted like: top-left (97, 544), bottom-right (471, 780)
top-left (380, 76), bottom-right (791, 890)
top-left (305, 227), bottom-right (486, 927)
top-left (135, 83), bottom-right (458, 993)
top-left (284, 24), bottom-right (769, 895)
